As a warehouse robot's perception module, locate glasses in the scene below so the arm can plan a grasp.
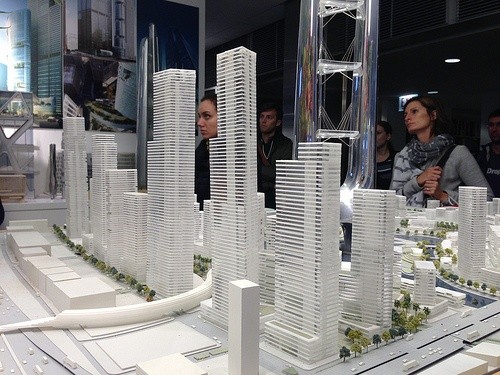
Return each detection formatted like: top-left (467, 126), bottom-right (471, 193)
top-left (489, 123), bottom-right (500, 127)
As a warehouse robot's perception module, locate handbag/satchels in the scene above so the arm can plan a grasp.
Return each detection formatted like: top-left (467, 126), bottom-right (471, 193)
top-left (423, 145), bottom-right (463, 208)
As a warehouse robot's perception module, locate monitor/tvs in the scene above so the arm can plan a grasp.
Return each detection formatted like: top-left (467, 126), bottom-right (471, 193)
top-left (399, 94), bottom-right (419, 112)
